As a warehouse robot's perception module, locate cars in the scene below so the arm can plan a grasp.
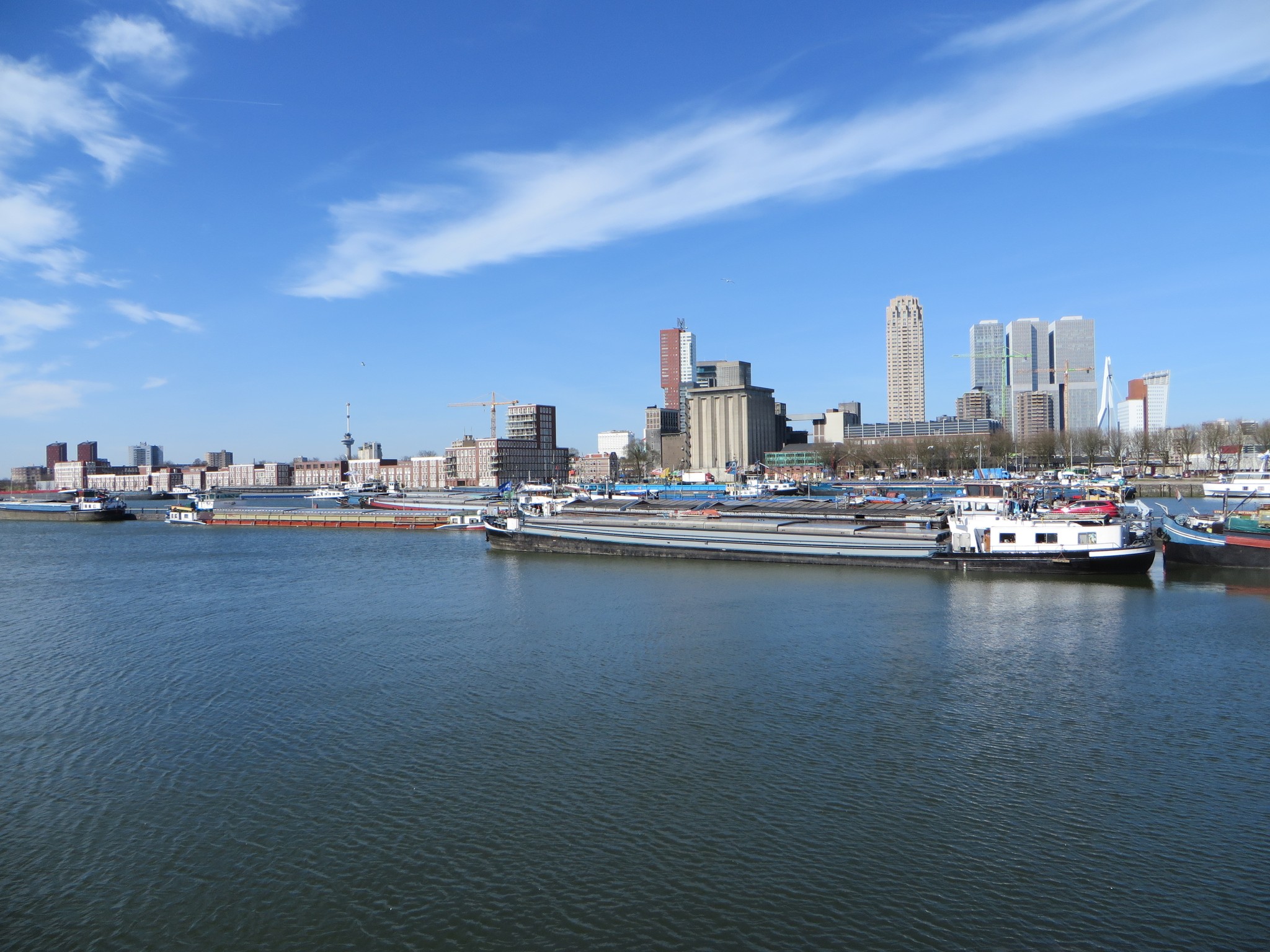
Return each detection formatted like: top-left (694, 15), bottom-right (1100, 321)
top-left (1209, 472), bottom-right (1222, 477)
top-left (1184, 473), bottom-right (1190, 478)
top-left (1152, 473), bottom-right (1167, 479)
top-left (1169, 473), bottom-right (1175, 478)
top-left (1175, 475), bottom-right (1182, 480)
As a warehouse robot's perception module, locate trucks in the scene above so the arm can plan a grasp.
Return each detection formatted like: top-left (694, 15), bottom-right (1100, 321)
top-left (1092, 465), bottom-right (1115, 477)
top-left (974, 467), bottom-right (1011, 480)
top-left (1111, 467), bottom-right (1136, 479)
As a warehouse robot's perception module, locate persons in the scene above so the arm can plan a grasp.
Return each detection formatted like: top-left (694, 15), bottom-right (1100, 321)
top-left (1010, 538), bottom-right (1014, 543)
top-left (1080, 503), bottom-right (1088, 507)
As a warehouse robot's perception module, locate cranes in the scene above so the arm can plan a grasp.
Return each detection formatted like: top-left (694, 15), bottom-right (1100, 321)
top-left (950, 346), bottom-right (1032, 438)
top-left (1013, 359), bottom-right (1096, 456)
top-left (447, 392), bottom-right (519, 438)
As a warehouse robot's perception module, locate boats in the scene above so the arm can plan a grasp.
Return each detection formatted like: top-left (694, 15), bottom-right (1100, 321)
top-left (305, 482), bottom-right (351, 502)
top-left (1155, 487), bottom-right (1270, 570)
top-left (0, 485), bottom-right (244, 525)
top-left (1202, 449), bottom-right (1270, 498)
top-left (336, 438), bottom-right (1156, 567)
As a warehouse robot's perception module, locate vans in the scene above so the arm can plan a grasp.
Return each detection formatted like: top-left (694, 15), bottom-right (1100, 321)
top-left (870, 475), bottom-right (884, 481)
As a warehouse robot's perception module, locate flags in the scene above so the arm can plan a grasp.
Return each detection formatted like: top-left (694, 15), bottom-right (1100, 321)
top-left (650, 468), bottom-right (662, 475)
top-left (677, 471), bottom-right (682, 476)
top-left (724, 462), bottom-right (736, 475)
top-left (501, 489), bottom-right (513, 498)
top-left (1175, 488), bottom-right (1184, 502)
top-left (659, 467), bottom-right (669, 477)
top-left (670, 469), bottom-right (678, 475)
top-left (498, 481), bottom-right (511, 490)
